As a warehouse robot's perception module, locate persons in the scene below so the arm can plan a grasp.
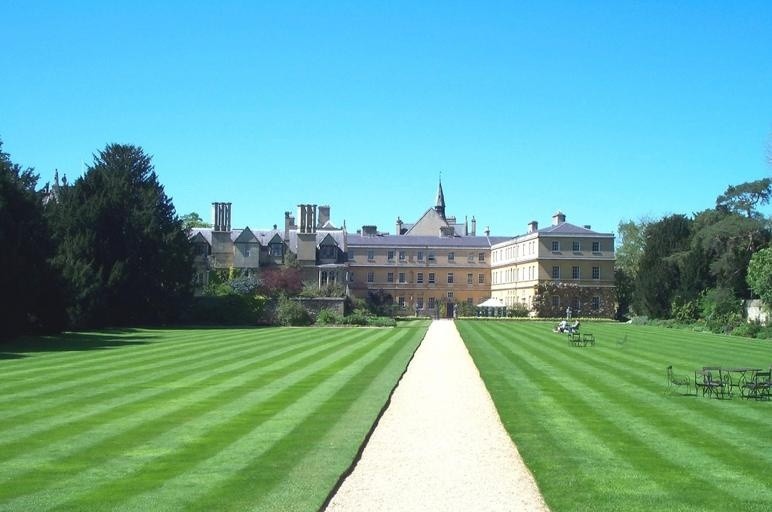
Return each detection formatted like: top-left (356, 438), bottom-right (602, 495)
top-left (568, 319), bottom-right (580, 335)
top-left (558, 318), bottom-right (568, 334)
top-left (565, 306), bottom-right (573, 322)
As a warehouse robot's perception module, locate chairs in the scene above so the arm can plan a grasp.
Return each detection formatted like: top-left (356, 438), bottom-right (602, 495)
top-left (665, 365), bottom-right (772, 403)
top-left (555, 323), bottom-right (595, 347)
